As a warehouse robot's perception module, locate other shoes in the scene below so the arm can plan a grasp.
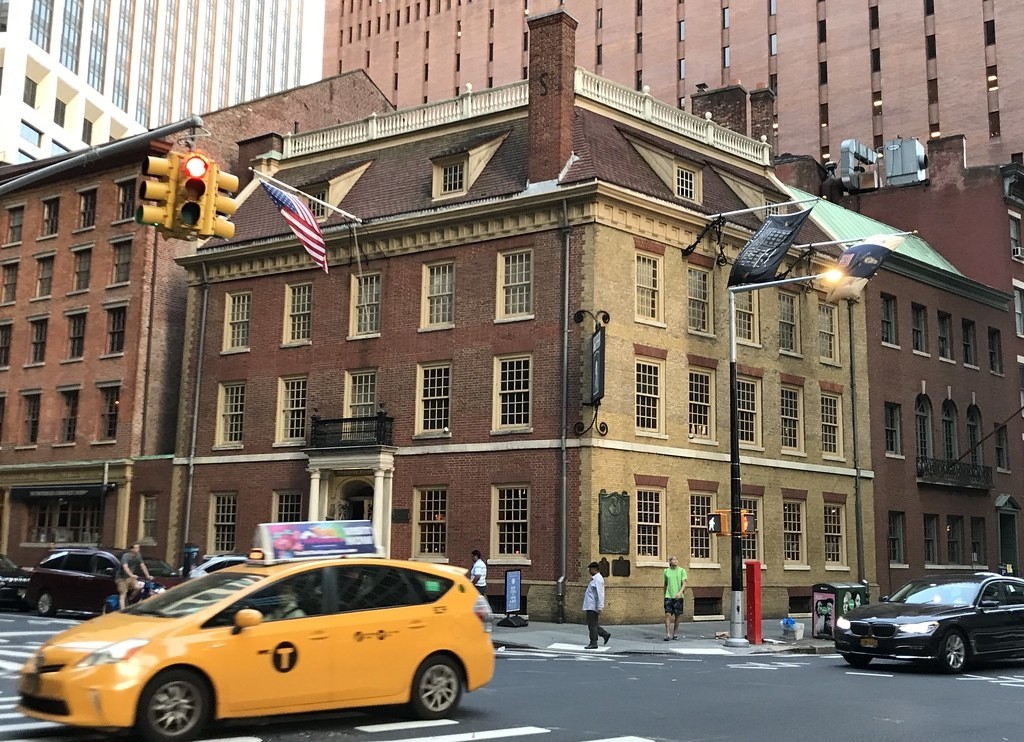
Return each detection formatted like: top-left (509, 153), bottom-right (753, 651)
top-left (603, 632), bottom-right (611, 644)
top-left (673, 634), bottom-right (679, 640)
top-left (584, 644), bottom-right (598, 649)
top-left (664, 635), bottom-right (670, 641)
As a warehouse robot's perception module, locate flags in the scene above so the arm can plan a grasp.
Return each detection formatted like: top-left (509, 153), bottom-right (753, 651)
top-left (258, 178), bottom-right (329, 275)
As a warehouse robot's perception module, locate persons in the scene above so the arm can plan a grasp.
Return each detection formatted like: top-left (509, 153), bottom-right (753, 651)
top-left (663, 556), bottom-right (687, 641)
top-left (471, 550), bottom-right (487, 595)
top-left (582, 562), bottom-right (610, 649)
top-left (115, 542), bottom-right (154, 610)
top-left (262, 584), bottom-right (305, 622)
top-left (814, 591), bottom-right (862, 638)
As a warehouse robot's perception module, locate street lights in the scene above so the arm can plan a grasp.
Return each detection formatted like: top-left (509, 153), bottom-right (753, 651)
top-left (724, 270), bottom-right (844, 649)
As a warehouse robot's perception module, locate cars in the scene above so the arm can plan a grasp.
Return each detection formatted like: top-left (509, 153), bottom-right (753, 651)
top-left (834, 571), bottom-right (1024, 675)
top-left (189, 553), bottom-right (248, 579)
top-left (0, 554), bottom-right (32, 610)
top-left (140, 557), bottom-right (178, 576)
top-left (15, 520), bottom-right (496, 742)
top-left (27, 548), bottom-right (166, 618)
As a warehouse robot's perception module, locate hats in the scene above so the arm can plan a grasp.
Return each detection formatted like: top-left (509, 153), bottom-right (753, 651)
top-left (588, 562), bottom-right (599, 568)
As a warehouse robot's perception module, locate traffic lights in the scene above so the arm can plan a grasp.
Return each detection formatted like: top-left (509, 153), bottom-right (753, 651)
top-left (741, 513), bottom-right (756, 534)
top-left (707, 513), bottom-right (728, 535)
top-left (197, 165), bottom-right (239, 241)
top-left (173, 155), bottom-right (210, 231)
top-left (134, 154), bottom-right (180, 231)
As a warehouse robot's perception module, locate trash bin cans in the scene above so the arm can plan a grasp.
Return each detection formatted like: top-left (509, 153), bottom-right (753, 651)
top-left (812, 583), bottom-right (868, 640)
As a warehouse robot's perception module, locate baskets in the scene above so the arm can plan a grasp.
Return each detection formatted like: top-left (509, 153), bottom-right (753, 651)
top-left (145, 582), bottom-right (166, 597)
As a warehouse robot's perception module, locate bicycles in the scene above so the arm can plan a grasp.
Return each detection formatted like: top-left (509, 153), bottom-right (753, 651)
top-left (102, 577), bottom-right (156, 616)
